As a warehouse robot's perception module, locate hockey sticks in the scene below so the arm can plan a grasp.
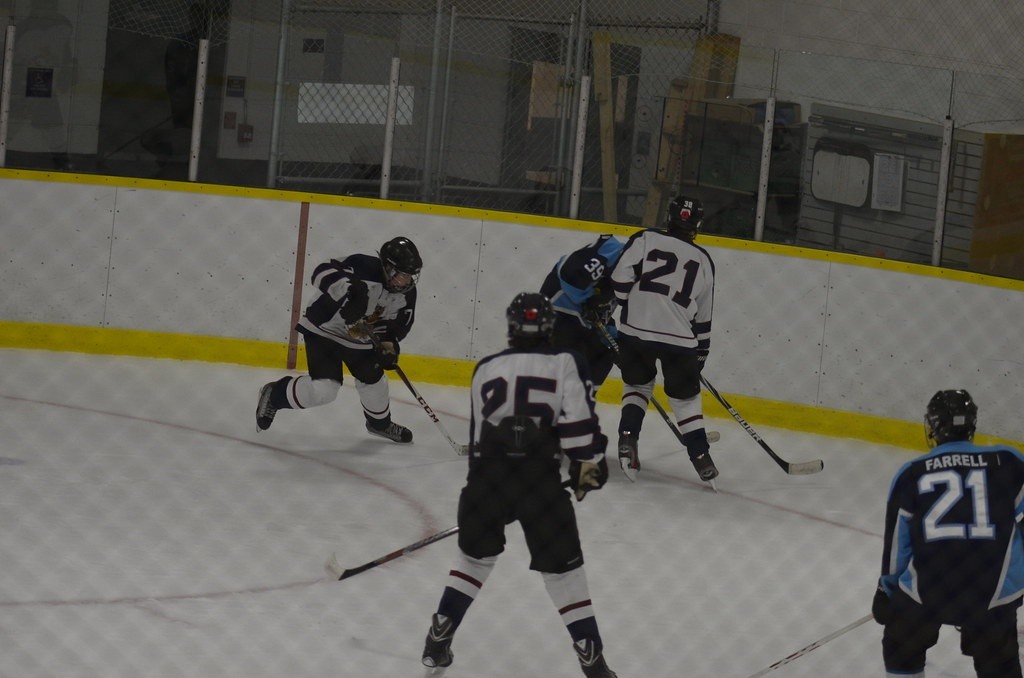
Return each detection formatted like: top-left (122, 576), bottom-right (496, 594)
top-left (325, 468), bottom-right (601, 581)
top-left (749, 612), bottom-right (874, 678)
top-left (364, 320), bottom-right (480, 455)
top-left (700, 374), bottom-right (824, 476)
top-left (597, 320), bottom-right (721, 446)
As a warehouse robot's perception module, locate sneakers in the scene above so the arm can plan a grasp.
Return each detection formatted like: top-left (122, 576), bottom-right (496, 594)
top-left (572, 638), bottom-right (618, 678)
top-left (420, 613), bottom-right (455, 678)
top-left (254, 381), bottom-right (277, 434)
top-left (363, 412), bottom-right (415, 443)
top-left (690, 450), bottom-right (719, 493)
top-left (618, 435), bottom-right (641, 483)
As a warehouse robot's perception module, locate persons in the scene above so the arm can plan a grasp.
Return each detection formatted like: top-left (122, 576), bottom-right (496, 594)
top-left (871, 388), bottom-right (1024, 678)
top-left (538, 233), bottom-right (625, 398)
top-left (608, 195), bottom-right (719, 482)
top-left (422, 291), bottom-right (617, 678)
top-left (255, 237), bottom-right (421, 439)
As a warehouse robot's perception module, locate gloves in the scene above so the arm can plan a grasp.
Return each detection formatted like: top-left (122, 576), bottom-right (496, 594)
top-left (581, 295), bottom-right (610, 328)
top-left (337, 298), bottom-right (372, 340)
top-left (698, 349), bottom-right (708, 373)
top-left (569, 456), bottom-right (608, 502)
top-left (372, 335), bottom-right (402, 373)
top-left (871, 588), bottom-right (891, 624)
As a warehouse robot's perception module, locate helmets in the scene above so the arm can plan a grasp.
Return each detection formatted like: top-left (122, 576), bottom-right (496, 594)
top-left (667, 193), bottom-right (705, 234)
top-left (505, 292), bottom-right (558, 347)
top-left (380, 237), bottom-right (424, 293)
top-left (927, 388), bottom-right (978, 444)
